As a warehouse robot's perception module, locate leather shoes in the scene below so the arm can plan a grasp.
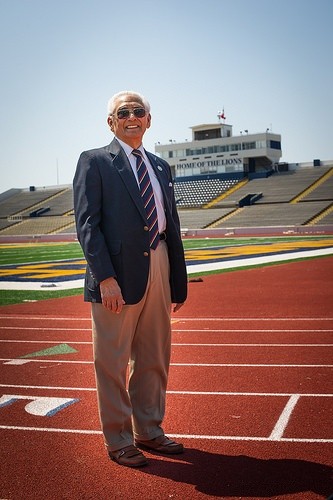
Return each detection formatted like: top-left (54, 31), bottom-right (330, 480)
top-left (135, 435), bottom-right (184, 454)
top-left (108, 445), bottom-right (149, 466)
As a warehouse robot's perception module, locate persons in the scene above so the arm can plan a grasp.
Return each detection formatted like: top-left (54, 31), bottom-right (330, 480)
top-left (73, 92), bottom-right (187, 466)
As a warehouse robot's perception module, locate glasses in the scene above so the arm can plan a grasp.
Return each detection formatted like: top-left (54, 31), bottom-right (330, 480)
top-left (112, 109), bottom-right (147, 119)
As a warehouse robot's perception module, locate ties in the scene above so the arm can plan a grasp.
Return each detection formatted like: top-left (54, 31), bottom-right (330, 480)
top-left (130, 149), bottom-right (160, 250)
